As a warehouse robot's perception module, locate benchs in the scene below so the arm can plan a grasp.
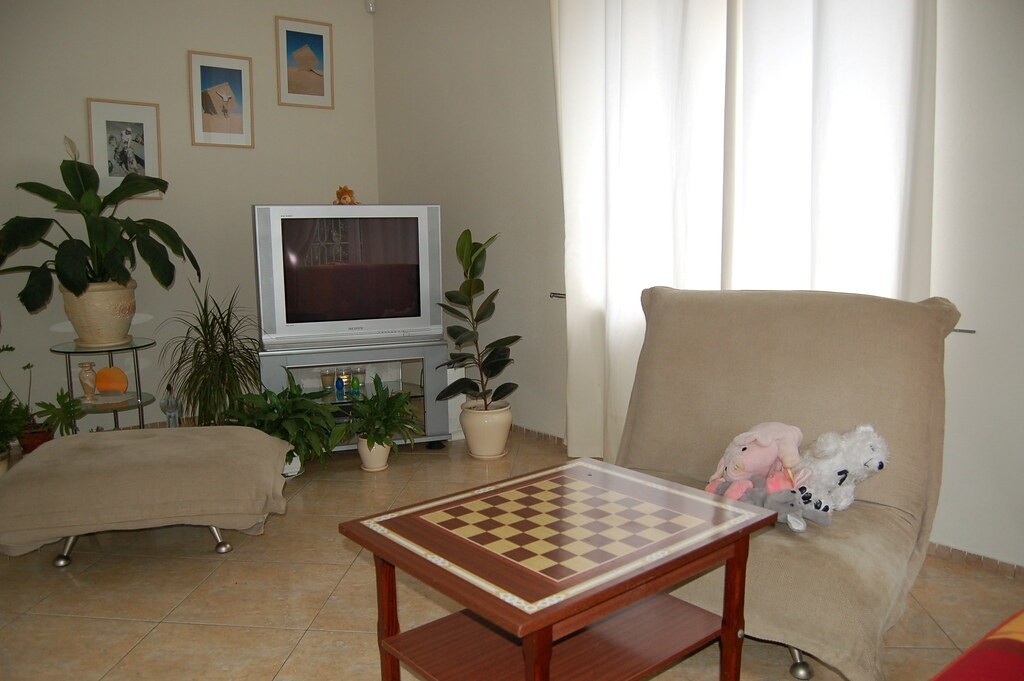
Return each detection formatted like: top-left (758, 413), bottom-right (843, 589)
top-left (0, 426), bottom-right (295, 568)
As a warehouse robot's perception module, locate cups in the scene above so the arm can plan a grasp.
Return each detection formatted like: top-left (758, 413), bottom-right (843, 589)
top-left (320, 370), bottom-right (335, 389)
top-left (352, 368), bottom-right (366, 387)
top-left (336, 367), bottom-right (351, 385)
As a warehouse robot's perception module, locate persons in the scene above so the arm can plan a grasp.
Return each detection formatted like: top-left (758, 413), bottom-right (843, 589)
top-left (216, 90), bottom-right (233, 121)
top-left (120, 127), bottom-right (133, 148)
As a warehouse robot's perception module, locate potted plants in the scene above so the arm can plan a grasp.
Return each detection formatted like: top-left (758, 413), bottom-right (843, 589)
top-left (0, 135), bottom-right (201, 347)
top-left (218, 362), bottom-right (339, 475)
top-left (436, 230), bottom-right (522, 460)
top-left (328, 372), bottom-right (425, 472)
top-left (0, 343), bottom-right (86, 477)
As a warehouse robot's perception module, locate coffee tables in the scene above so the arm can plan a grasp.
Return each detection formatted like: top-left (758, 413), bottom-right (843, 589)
top-left (337, 456), bottom-right (777, 681)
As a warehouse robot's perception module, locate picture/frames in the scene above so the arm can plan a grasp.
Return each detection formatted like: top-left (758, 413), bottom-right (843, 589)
top-left (187, 50), bottom-right (256, 148)
top-left (87, 97), bottom-right (163, 200)
top-left (274, 15), bottom-right (336, 110)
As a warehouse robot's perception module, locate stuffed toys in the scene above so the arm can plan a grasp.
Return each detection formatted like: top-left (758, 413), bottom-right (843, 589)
top-left (709, 422), bottom-right (803, 483)
top-left (790, 424), bottom-right (889, 512)
top-left (336, 185), bottom-right (354, 205)
top-left (705, 470), bottom-right (831, 532)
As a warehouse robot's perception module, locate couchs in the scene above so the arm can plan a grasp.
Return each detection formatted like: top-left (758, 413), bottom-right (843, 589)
top-left (613, 286), bottom-right (961, 681)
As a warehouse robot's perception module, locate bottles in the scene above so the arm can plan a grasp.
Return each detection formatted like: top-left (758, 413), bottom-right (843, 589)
top-left (78, 362), bottom-right (97, 399)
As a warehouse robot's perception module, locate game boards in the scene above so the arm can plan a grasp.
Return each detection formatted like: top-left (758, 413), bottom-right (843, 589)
top-left (419, 474), bottom-right (706, 583)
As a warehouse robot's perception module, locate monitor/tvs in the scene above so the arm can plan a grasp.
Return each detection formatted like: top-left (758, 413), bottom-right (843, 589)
top-left (254, 203), bottom-right (444, 353)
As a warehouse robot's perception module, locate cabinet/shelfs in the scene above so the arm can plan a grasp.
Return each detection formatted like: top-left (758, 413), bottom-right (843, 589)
top-left (258, 337), bottom-right (453, 456)
top-left (49, 337), bottom-right (156, 436)
